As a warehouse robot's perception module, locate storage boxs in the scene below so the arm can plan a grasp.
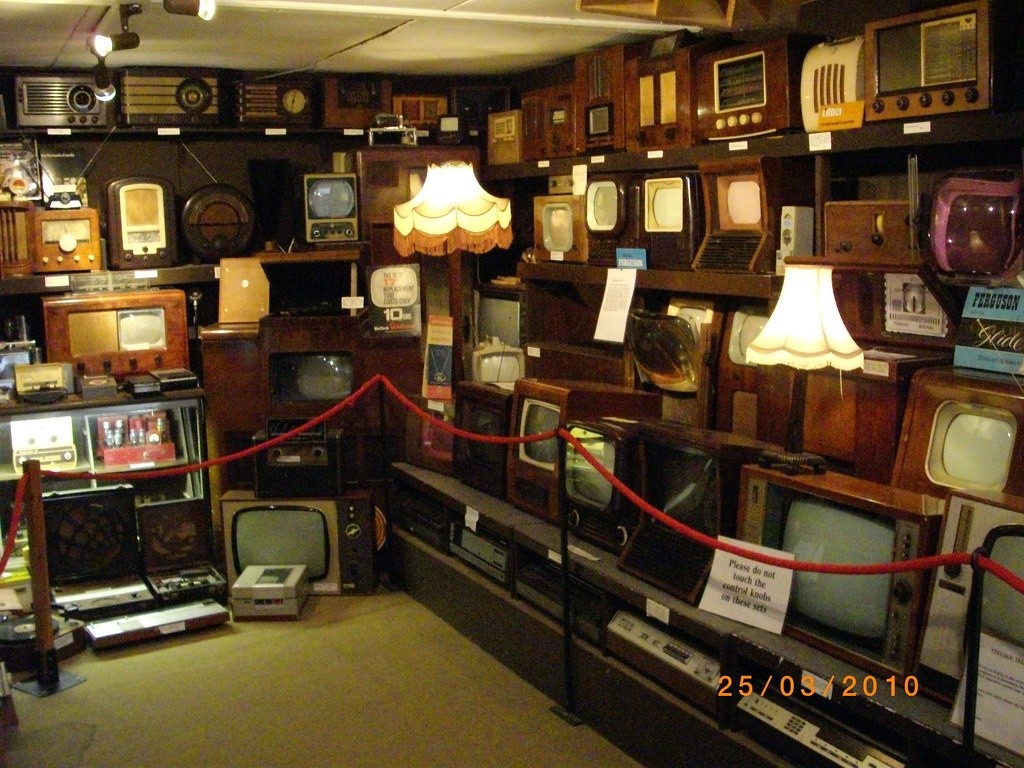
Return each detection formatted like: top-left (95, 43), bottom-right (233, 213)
top-left (232, 565), bottom-right (310, 617)
top-left (96, 410), bottom-right (177, 466)
top-left (42, 484), bottom-right (157, 623)
top-left (137, 497), bottom-right (227, 604)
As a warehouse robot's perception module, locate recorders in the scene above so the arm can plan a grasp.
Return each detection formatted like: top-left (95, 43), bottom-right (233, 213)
top-left (14, 70), bottom-right (315, 128)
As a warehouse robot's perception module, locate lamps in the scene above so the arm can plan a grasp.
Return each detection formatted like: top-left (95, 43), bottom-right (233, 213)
top-left (745, 255), bottom-right (866, 371)
top-left (88, 0), bottom-right (217, 102)
top-left (395, 159), bottom-right (514, 256)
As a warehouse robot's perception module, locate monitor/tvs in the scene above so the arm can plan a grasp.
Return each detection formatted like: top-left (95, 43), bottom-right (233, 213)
top-left (222, 168), bottom-right (1024, 711)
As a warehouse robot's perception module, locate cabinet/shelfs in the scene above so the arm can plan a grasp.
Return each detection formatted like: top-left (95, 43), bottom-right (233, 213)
top-left (0, 388), bottom-right (213, 561)
top-left (0, 125), bottom-right (486, 294)
top-left (488, 109), bottom-right (1024, 290)
top-left (388, 463), bottom-right (1024, 768)
